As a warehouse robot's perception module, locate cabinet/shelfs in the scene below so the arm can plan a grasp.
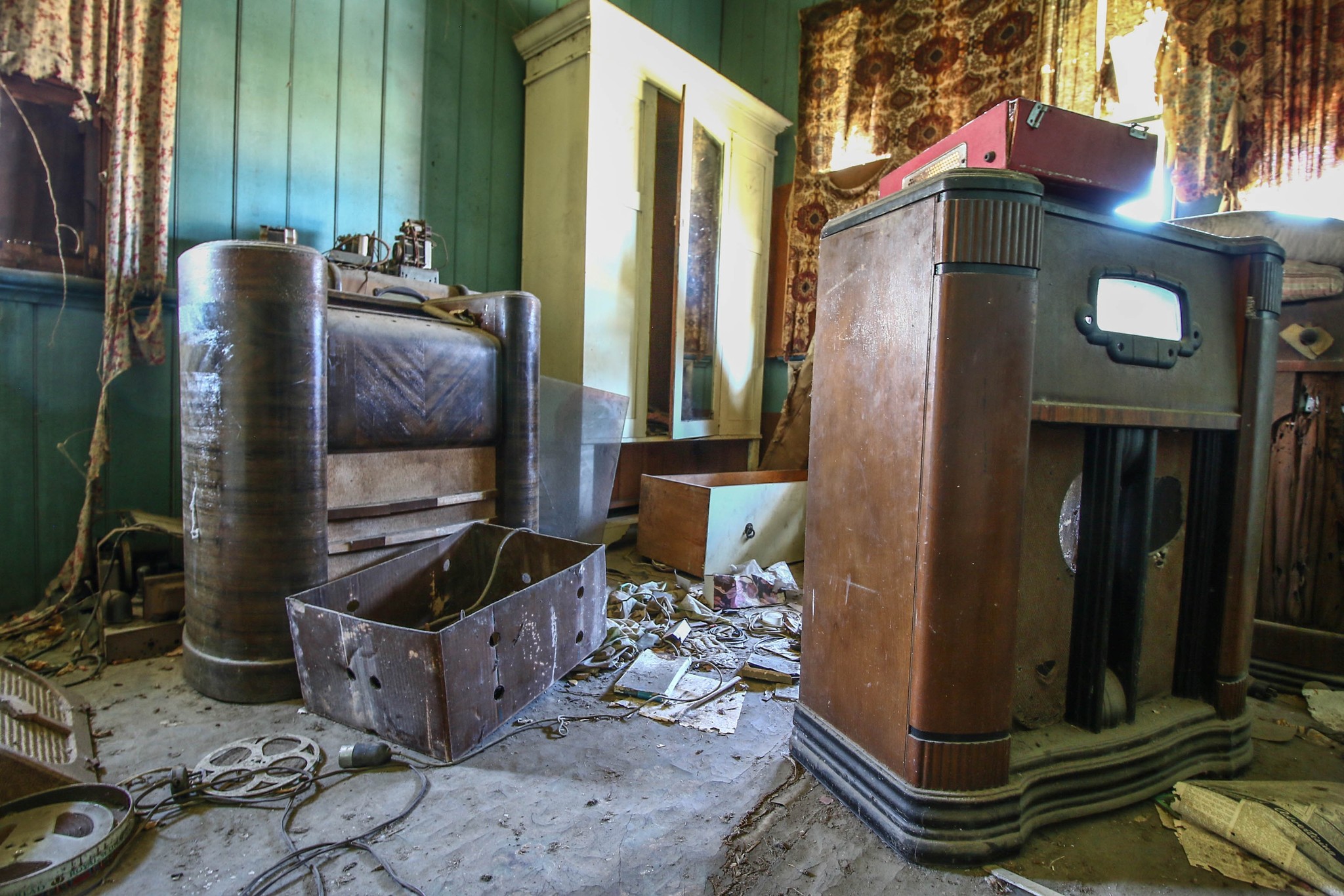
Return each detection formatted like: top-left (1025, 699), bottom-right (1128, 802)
top-left (514, 0), bottom-right (795, 524)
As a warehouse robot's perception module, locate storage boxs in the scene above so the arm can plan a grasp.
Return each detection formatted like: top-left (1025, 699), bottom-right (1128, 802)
top-left (879, 97), bottom-right (1158, 209)
top-left (286, 520), bottom-right (606, 762)
top-left (638, 470), bottom-right (808, 581)
